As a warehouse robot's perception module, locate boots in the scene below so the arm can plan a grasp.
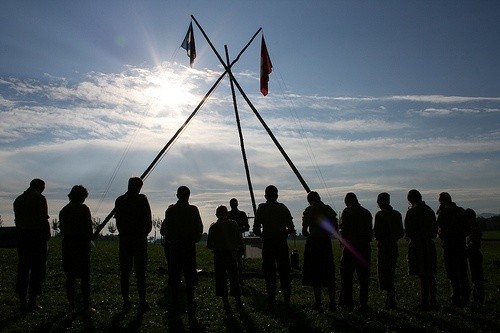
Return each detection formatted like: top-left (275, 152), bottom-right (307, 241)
top-left (17, 286), bottom-right (29, 314)
top-left (136, 279), bottom-right (151, 314)
top-left (28, 283), bottom-right (43, 313)
top-left (164, 287), bottom-right (181, 322)
top-left (118, 280), bottom-right (132, 316)
top-left (186, 289), bottom-right (209, 327)
top-left (66, 292), bottom-right (76, 314)
top-left (81, 287), bottom-right (93, 312)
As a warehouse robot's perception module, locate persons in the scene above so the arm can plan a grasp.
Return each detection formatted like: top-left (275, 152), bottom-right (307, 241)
top-left (437, 192), bottom-right (485, 304)
top-left (14, 180), bottom-right (51, 309)
top-left (340, 193), bottom-right (372, 309)
top-left (161, 186), bottom-right (248, 308)
top-left (405, 190), bottom-right (438, 305)
top-left (59, 186), bottom-right (97, 312)
top-left (253, 186), bottom-right (295, 305)
top-left (116, 178), bottom-right (152, 311)
top-left (303, 192), bottom-right (338, 309)
top-left (374, 193), bottom-right (403, 307)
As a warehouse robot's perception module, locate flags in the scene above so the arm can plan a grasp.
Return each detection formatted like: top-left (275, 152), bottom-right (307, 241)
top-left (181, 22), bottom-right (196, 67)
top-left (260, 31), bottom-right (273, 97)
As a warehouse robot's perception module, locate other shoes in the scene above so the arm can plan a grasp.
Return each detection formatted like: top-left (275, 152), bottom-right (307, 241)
top-left (413, 300), bottom-right (441, 312)
top-left (358, 302), bottom-right (368, 313)
top-left (267, 291), bottom-right (279, 305)
top-left (448, 296), bottom-right (470, 309)
top-left (221, 303), bottom-right (232, 311)
top-left (284, 295), bottom-right (293, 305)
top-left (473, 299), bottom-right (485, 311)
top-left (341, 301), bottom-right (354, 311)
top-left (379, 301), bottom-right (400, 310)
top-left (236, 302), bottom-right (246, 310)
top-left (328, 302), bottom-right (338, 313)
top-left (311, 301), bottom-right (326, 312)
